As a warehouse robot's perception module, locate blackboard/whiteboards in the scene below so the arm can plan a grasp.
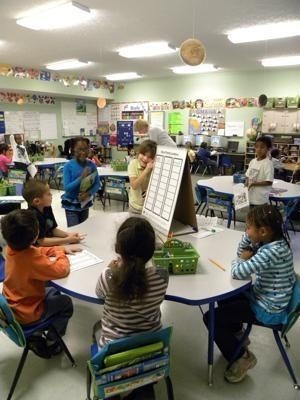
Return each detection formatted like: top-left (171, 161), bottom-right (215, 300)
top-left (151, 111), bottom-right (165, 130)
top-left (61, 101), bottom-right (97, 138)
top-left (110, 101), bottom-right (149, 130)
top-left (262, 110), bottom-right (300, 136)
top-left (188, 109), bottom-right (226, 136)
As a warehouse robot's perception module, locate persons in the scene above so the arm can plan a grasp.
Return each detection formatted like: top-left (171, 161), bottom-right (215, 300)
top-left (241, 135), bottom-right (274, 210)
top-left (0, 144), bottom-right (13, 179)
top-left (21, 178), bottom-right (88, 248)
top-left (9, 133), bottom-right (32, 180)
top-left (197, 142), bottom-right (219, 176)
top-left (127, 140), bottom-right (158, 218)
top-left (89, 216), bottom-right (170, 354)
top-left (133, 119), bottom-right (178, 148)
top-left (61, 136), bottom-right (100, 226)
top-left (1, 208), bottom-right (84, 360)
top-left (185, 140), bottom-right (196, 164)
top-left (271, 144), bottom-right (293, 179)
top-left (202, 203), bottom-right (297, 384)
top-left (84, 138), bottom-right (102, 167)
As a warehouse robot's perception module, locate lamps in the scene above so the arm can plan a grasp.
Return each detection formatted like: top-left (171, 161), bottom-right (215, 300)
top-left (171, 65), bottom-right (221, 75)
top-left (115, 40), bottom-right (180, 60)
top-left (16, 0), bottom-right (92, 32)
top-left (46, 55), bottom-right (89, 72)
top-left (106, 71), bottom-right (143, 83)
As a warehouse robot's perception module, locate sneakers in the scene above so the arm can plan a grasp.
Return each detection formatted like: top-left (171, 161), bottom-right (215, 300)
top-left (225, 337), bottom-right (257, 382)
top-left (26, 335), bottom-right (63, 359)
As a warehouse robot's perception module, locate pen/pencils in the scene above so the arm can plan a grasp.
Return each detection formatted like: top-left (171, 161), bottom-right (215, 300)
top-left (208, 258), bottom-right (226, 271)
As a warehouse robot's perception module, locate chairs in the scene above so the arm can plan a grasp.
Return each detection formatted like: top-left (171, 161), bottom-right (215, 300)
top-left (162, 195), bottom-right (171, 199)
top-left (0, 146), bottom-right (300, 400)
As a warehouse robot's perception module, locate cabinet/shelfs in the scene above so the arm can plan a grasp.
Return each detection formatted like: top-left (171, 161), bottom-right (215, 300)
top-left (244, 133), bottom-right (300, 174)
top-left (261, 106), bottom-right (300, 132)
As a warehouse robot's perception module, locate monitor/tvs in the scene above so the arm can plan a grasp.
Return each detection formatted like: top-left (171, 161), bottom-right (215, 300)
top-left (228, 141), bottom-right (238, 152)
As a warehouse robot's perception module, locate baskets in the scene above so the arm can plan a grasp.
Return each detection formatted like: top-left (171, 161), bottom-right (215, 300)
top-left (30, 153), bottom-right (44, 162)
top-left (0, 177), bottom-right (24, 196)
top-left (110, 160), bottom-right (127, 171)
top-left (153, 239), bottom-right (199, 275)
